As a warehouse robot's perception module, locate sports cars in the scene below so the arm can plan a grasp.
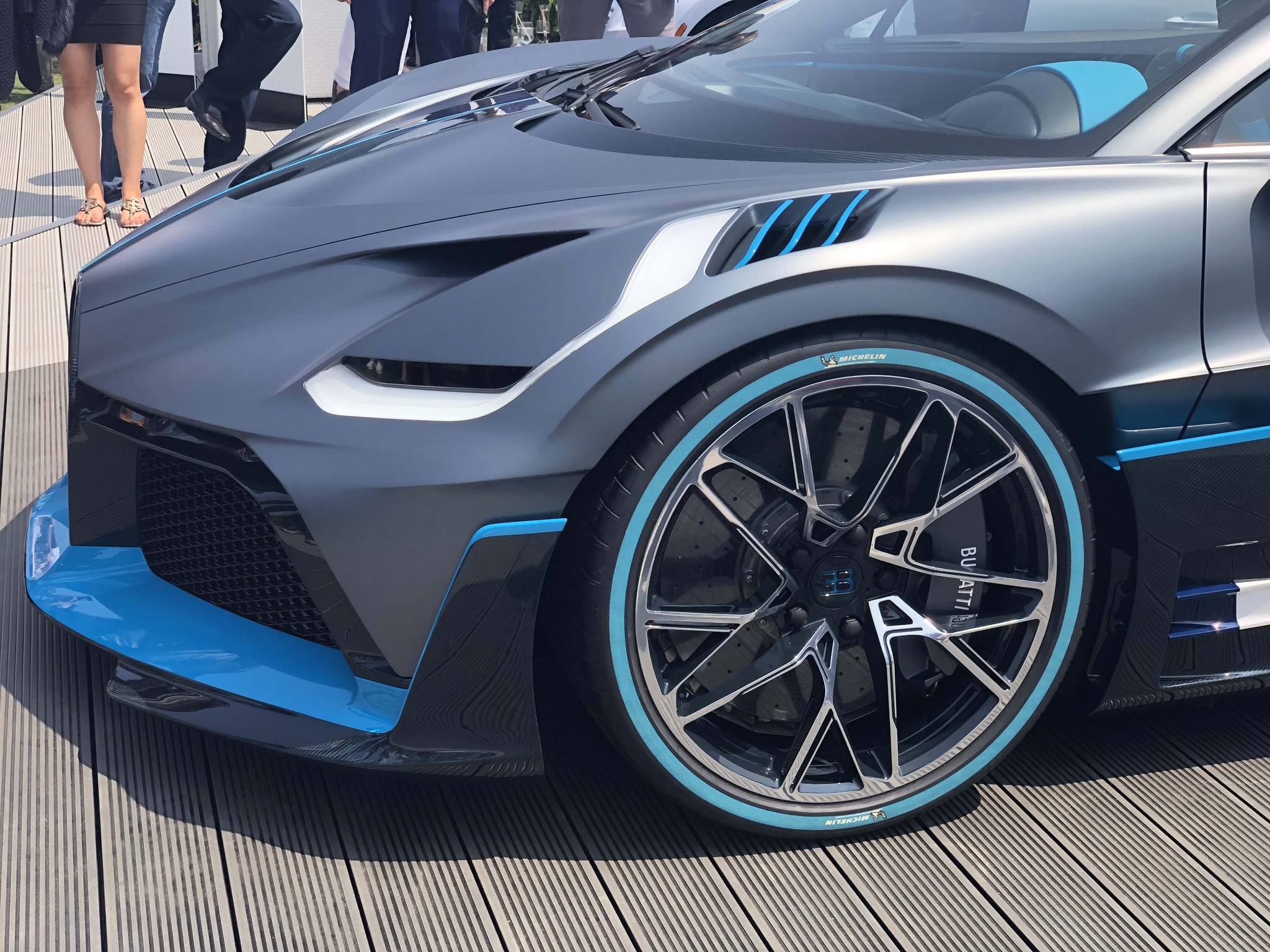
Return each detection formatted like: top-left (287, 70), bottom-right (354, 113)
top-left (23, 0), bottom-right (1270, 842)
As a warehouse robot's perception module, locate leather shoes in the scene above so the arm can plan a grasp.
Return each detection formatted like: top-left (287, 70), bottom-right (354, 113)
top-left (184, 87), bottom-right (231, 143)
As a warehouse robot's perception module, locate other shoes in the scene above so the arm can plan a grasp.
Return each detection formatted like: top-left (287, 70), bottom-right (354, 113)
top-left (332, 78), bottom-right (351, 105)
top-left (101, 169), bottom-right (156, 204)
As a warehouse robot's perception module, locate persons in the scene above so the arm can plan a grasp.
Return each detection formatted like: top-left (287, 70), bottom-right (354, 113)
top-left (0, 0), bottom-right (674, 228)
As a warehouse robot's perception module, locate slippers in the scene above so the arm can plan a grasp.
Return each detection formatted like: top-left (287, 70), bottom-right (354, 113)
top-left (75, 196), bottom-right (111, 226)
top-left (120, 197), bottom-right (149, 228)
